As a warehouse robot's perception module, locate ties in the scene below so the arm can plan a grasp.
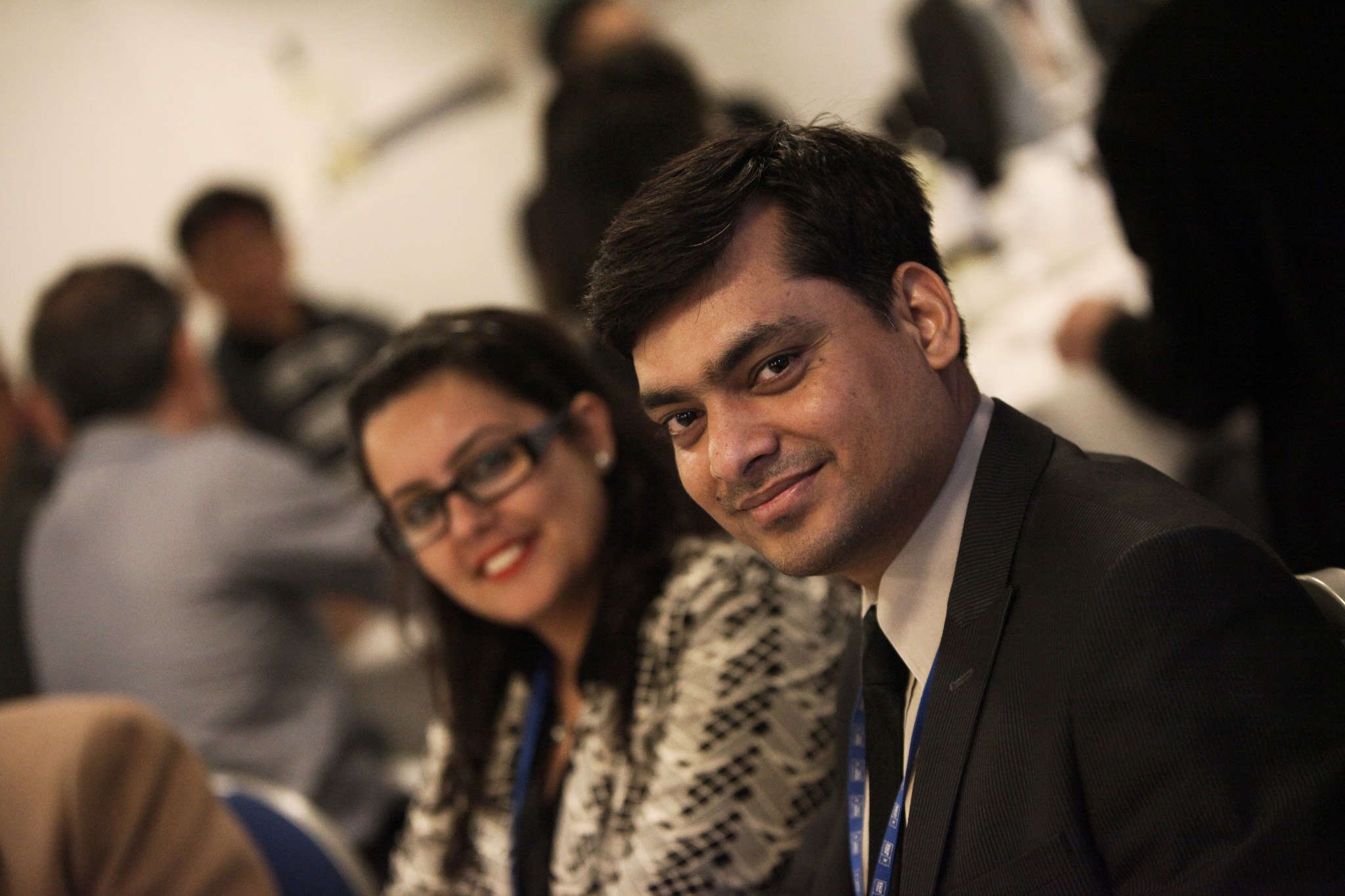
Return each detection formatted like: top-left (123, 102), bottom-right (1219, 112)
top-left (860, 604), bottom-right (909, 896)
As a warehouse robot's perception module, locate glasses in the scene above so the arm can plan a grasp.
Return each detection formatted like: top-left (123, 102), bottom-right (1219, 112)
top-left (372, 411), bottom-right (577, 561)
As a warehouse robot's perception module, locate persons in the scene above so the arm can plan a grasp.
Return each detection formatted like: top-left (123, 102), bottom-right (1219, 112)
top-left (0, 692), bottom-right (280, 896)
top-left (342, 304), bottom-right (865, 896)
top-left (174, 186), bottom-right (393, 469)
top-left (19, 261), bottom-right (446, 883)
top-left (0, 342), bottom-right (62, 707)
top-left (522, 0), bottom-right (1345, 575)
top-left (581, 115), bottom-right (1345, 896)
